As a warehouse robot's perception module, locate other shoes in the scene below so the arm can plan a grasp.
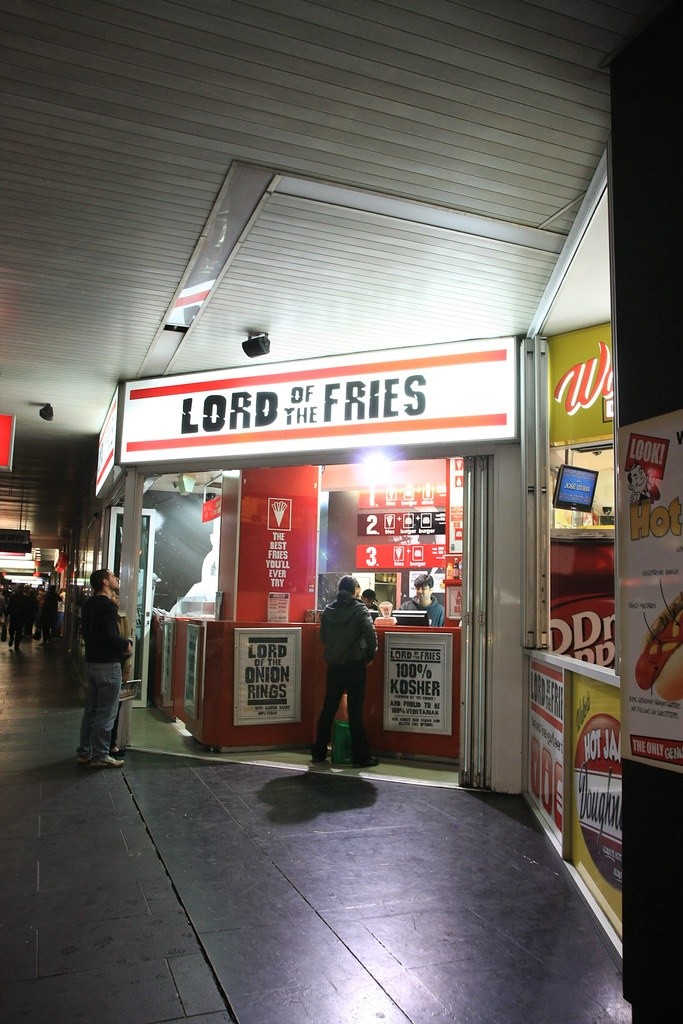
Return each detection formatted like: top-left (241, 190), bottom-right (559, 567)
top-left (354, 757), bottom-right (379, 767)
top-left (9, 636), bottom-right (14, 647)
top-left (110, 748), bottom-right (126, 757)
top-left (77, 756), bottom-right (92, 762)
top-left (86, 756), bottom-right (125, 768)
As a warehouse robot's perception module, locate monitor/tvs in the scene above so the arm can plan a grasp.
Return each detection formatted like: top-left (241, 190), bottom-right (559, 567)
top-left (390, 610), bottom-right (428, 626)
top-left (552, 464), bottom-right (598, 513)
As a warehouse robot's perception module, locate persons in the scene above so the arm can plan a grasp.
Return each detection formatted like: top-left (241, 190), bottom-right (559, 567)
top-left (308, 574), bottom-right (380, 767)
top-left (74, 569), bottom-right (131, 768)
top-left (0, 583), bottom-right (75, 650)
top-left (399, 574), bottom-right (446, 627)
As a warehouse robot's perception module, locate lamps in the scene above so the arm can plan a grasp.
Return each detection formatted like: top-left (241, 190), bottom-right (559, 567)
top-left (40, 406), bottom-right (53, 421)
top-left (242, 337), bottom-right (270, 358)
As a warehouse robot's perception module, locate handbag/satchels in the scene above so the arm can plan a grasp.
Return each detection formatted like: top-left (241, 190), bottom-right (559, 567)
top-left (33, 628), bottom-right (41, 640)
top-left (1, 623), bottom-right (6, 642)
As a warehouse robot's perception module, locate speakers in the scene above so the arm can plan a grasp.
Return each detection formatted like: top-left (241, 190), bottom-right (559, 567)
top-left (40, 406), bottom-right (53, 421)
top-left (242, 336), bottom-right (271, 357)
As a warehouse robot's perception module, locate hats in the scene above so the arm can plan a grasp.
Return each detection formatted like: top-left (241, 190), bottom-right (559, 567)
top-left (363, 589), bottom-right (379, 603)
